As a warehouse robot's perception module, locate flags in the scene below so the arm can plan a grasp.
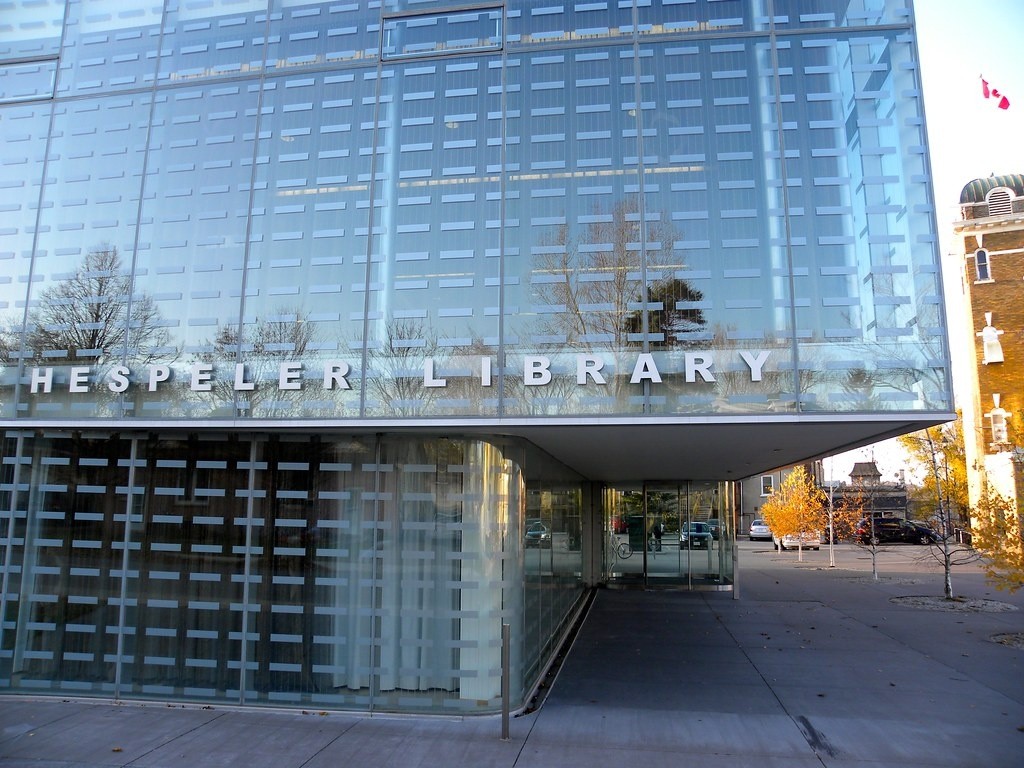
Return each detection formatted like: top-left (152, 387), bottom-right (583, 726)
top-left (982, 80), bottom-right (1010, 109)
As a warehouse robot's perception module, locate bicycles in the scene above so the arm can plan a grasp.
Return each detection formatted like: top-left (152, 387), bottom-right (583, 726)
top-left (615, 537), bottom-right (633, 560)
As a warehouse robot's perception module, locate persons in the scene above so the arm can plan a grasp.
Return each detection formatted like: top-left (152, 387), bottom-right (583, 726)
top-left (647, 518), bottom-right (661, 550)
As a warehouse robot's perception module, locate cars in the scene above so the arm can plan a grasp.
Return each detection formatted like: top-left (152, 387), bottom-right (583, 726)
top-left (772, 527), bottom-right (821, 550)
top-left (706, 519), bottom-right (728, 541)
top-left (852, 517), bottom-right (937, 545)
top-left (525, 523), bottom-right (552, 549)
top-left (748, 519), bottom-right (772, 541)
top-left (676, 520), bottom-right (713, 550)
